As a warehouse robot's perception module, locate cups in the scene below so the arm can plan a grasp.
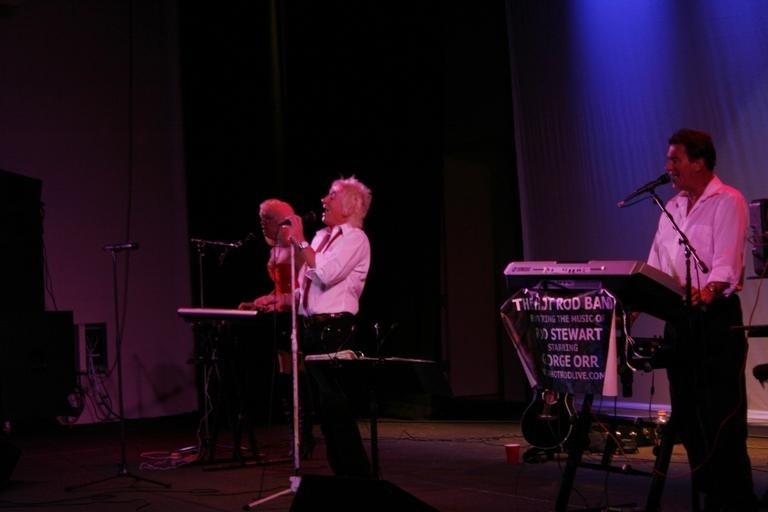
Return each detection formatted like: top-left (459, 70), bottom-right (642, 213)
top-left (505, 444), bottom-right (521, 464)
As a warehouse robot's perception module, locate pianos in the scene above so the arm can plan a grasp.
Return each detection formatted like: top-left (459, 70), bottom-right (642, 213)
top-left (177, 307), bottom-right (266, 327)
top-left (503, 260), bottom-right (706, 313)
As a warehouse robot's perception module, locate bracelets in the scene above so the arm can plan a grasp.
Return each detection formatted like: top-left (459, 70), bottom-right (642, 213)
top-left (299, 239), bottom-right (310, 250)
top-left (706, 281), bottom-right (718, 294)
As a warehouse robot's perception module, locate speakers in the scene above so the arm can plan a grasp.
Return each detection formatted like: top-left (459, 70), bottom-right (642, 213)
top-left (289, 474), bottom-right (441, 512)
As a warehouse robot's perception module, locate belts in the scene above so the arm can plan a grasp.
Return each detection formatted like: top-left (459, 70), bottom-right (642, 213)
top-left (308, 313), bottom-right (346, 322)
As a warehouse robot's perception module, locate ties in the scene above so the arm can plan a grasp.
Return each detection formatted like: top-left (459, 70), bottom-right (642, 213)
top-left (301, 229), bottom-right (332, 310)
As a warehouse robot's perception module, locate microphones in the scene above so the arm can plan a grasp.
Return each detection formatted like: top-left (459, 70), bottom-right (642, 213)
top-left (282, 210), bottom-right (317, 226)
top-left (616, 171), bottom-right (674, 208)
top-left (187, 236), bottom-right (241, 257)
top-left (101, 241), bottom-right (138, 250)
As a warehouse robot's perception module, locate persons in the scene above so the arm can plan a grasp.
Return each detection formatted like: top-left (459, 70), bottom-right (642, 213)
top-left (283, 175), bottom-right (373, 477)
top-left (237, 199), bottom-right (318, 462)
top-left (643, 127), bottom-right (763, 512)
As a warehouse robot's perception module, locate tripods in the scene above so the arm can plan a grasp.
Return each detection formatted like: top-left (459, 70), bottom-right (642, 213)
top-left (242, 227), bottom-right (302, 512)
top-left (160, 238), bottom-right (267, 467)
top-left (65, 251), bottom-right (171, 493)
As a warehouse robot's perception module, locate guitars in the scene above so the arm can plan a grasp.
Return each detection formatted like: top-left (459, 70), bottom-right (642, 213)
top-left (520, 384), bottom-right (579, 450)
